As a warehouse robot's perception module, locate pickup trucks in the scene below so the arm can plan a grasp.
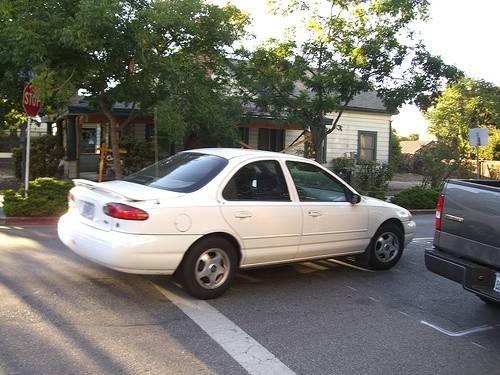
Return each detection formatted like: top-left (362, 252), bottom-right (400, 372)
top-left (424, 176), bottom-right (500, 308)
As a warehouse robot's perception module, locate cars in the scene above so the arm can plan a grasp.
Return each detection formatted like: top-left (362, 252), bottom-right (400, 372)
top-left (56, 147), bottom-right (416, 300)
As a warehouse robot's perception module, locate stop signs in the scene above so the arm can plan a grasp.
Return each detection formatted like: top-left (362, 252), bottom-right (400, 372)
top-left (23, 83), bottom-right (41, 116)
